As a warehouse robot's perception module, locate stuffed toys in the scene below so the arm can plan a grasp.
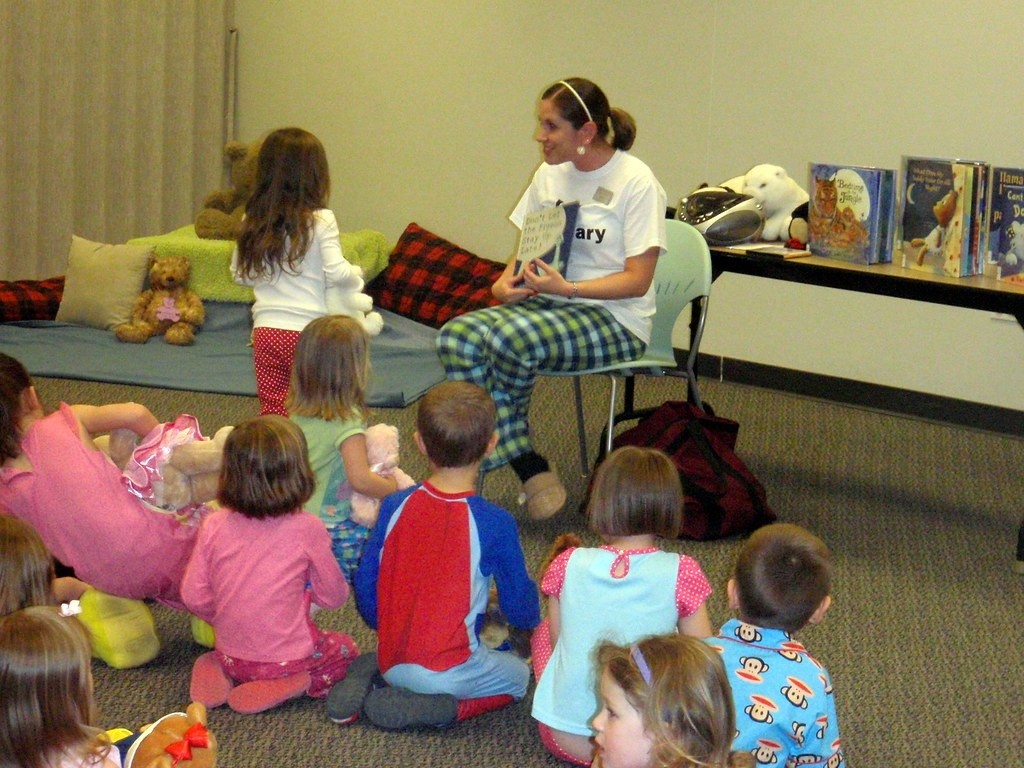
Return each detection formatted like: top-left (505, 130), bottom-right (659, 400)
top-left (94, 701), bottom-right (218, 768)
top-left (91, 413), bottom-right (235, 510)
top-left (102, 243), bottom-right (204, 347)
top-left (349, 423), bottom-right (417, 532)
top-left (740, 163), bottom-right (810, 247)
top-left (52, 577), bottom-right (161, 670)
top-left (324, 264), bottom-right (386, 337)
top-left (194, 140), bottom-right (259, 242)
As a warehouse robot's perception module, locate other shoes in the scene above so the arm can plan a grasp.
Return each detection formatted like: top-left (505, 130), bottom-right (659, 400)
top-left (519, 462), bottom-right (566, 520)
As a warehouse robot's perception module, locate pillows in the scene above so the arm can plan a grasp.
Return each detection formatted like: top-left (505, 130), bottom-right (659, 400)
top-left (361, 222), bottom-right (524, 330)
top-left (55, 231), bottom-right (158, 330)
top-left (0, 276), bottom-right (65, 322)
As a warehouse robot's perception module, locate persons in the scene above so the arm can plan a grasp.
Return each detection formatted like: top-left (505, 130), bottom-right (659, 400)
top-left (0, 352), bottom-right (850, 768)
top-left (436, 78), bottom-right (668, 520)
top-left (231, 127), bottom-right (359, 411)
top-left (283, 314), bottom-right (398, 583)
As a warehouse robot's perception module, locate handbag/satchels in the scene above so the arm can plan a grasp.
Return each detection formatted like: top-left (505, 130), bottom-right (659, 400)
top-left (584, 400), bottom-right (779, 542)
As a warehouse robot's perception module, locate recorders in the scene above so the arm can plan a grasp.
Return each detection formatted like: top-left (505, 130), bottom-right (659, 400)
top-left (676, 187), bottom-right (766, 245)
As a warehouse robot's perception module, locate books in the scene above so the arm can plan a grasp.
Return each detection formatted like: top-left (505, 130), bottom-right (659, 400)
top-left (988, 167), bottom-right (1024, 283)
top-left (808, 162), bottom-right (895, 264)
top-left (512, 201), bottom-right (580, 286)
top-left (897, 154), bottom-right (990, 278)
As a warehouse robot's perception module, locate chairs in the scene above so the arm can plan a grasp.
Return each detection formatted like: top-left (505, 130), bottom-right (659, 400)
top-left (535, 218), bottom-right (712, 479)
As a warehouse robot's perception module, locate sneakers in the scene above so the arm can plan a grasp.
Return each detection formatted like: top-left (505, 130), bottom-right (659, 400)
top-left (190, 655), bottom-right (231, 708)
top-left (363, 688), bottom-right (458, 729)
top-left (228, 672), bottom-right (311, 713)
top-left (326, 652), bottom-right (379, 725)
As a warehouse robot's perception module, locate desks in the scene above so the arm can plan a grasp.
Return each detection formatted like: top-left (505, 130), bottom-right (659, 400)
top-left (597, 240), bottom-right (1024, 460)
